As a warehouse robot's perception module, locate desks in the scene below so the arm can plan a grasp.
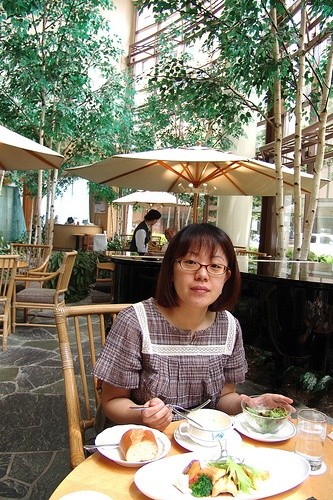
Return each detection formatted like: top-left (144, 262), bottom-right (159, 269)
top-left (0, 259), bottom-right (29, 334)
top-left (49, 419), bottom-right (333, 500)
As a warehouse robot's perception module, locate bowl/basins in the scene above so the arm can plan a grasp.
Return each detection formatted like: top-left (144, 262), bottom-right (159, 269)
top-left (240, 394), bottom-right (292, 434)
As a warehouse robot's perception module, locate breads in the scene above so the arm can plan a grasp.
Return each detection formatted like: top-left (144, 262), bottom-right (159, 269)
top-left (119, 428), bottom-right (158, 462)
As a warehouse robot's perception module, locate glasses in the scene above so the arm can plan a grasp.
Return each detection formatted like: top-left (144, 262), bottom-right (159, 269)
top-left (175, 258), bottom-right (230, 275)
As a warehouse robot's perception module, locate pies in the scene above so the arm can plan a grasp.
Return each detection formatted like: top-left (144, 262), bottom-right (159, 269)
top-left (204, 466), bottom-right (238, 497)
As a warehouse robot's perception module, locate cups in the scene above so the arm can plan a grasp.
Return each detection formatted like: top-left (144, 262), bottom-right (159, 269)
top-left (293, 408), bottom-right (328, 471)
top-left (179, 408), bottom-right (232, 447)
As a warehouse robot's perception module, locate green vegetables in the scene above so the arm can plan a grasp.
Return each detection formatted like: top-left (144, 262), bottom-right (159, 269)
top-left (189, 474), bottom-right (212, 496)
top-left (258, 407), bottom-right (285, 433)
top-left (208, 457), bottom-right (259, 492)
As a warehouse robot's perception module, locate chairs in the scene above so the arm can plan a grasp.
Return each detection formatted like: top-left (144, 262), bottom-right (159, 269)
top-left (54, 303), bottom-right (134, 473)
top-left (86, 233), bottom-right (115, 303)
top-left (0, 243), bottom-right (77, 351)
top-left (240, 251), bottom-right (267, 257)
top-left (73, 234), bottom-right (85, 250)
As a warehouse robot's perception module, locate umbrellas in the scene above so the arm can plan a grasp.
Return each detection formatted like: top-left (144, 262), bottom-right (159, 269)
top-left (64, 142), bottom-right (330, 223)
top-left (113, 189), bottom-right (190, 209)
top-left (0, 125), bottom-right (65, 171)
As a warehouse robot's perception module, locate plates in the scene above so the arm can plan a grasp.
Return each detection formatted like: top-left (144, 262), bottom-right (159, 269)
top-left (233, 413), bottom-right (297, 443)
top-left (57, 490), bottom-right (113, 500)
top-left (134, 444), bottom-right (311, 500)
top-left (173, 422), bottom-right (242, 452)
top-left (94, 424), bottom-right (171, 466)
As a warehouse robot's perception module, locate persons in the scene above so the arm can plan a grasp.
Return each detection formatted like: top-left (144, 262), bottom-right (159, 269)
top-left (162, 227), bottom-right (177, 253)
top-left (129, 209), bottom-right (161, 252)
top-left (91, 223), bottom-right (296, 432)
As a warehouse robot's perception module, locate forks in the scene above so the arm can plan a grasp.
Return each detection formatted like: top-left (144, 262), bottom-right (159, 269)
top-left (130, 398), bottom-right (212, 411)
